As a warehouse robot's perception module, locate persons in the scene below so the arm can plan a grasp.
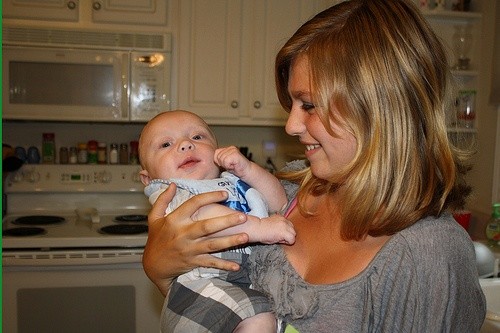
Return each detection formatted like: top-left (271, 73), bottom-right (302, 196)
top-left (139, 110), bottom-right (297, 333)
top-left (142, 0), bottom-right (488, 333)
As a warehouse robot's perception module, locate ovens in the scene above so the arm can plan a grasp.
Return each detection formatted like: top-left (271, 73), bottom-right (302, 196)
top-left (2, 249), bottom-right (168, 333)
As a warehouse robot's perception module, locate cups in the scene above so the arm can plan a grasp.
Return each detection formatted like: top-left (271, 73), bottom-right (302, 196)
top-left (457, 89), bottom-right (475, 130)
top-left (451, 210), bottom-right (471, 232)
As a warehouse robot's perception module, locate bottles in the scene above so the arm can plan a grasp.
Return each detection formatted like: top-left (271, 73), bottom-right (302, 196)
top-left (452, 23), bottom-right (472, 71)
top-left (108, 143), bottom-right (128, 165)
top-left (485, 204), bottom-right (500, 247)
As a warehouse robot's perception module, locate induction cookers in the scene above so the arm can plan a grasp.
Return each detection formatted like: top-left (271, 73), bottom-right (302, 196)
top-left (2, 164), bottom-right (149, 250)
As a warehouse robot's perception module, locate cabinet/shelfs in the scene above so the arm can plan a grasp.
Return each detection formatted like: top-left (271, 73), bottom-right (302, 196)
top-left (1, 0), bottom-right (172, 35)
top-left (172, 0), bottom-right (339, 127)
